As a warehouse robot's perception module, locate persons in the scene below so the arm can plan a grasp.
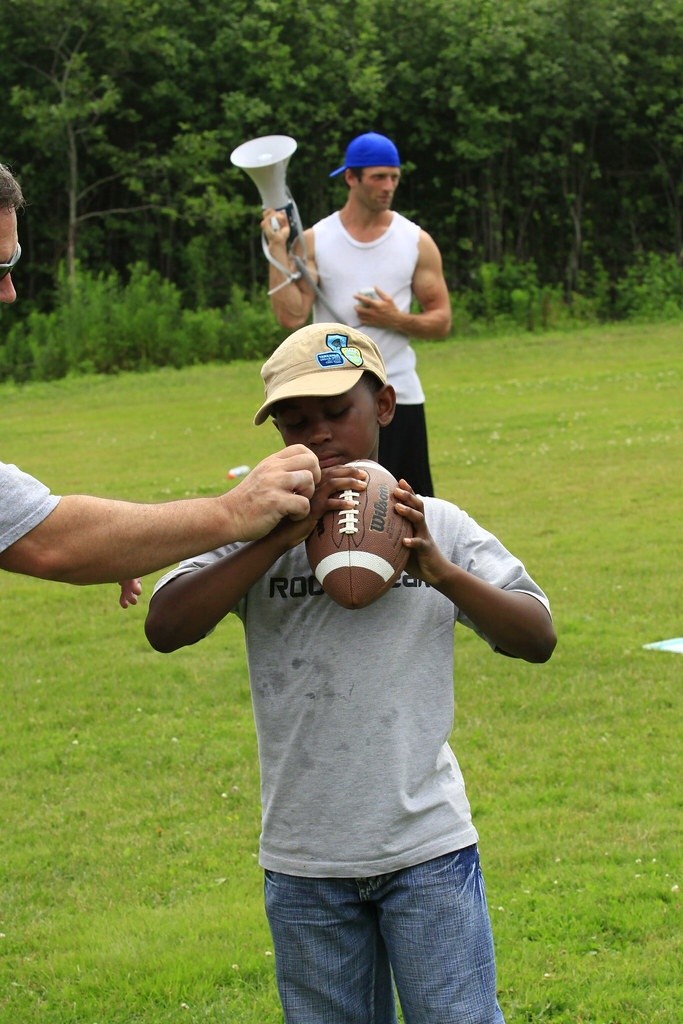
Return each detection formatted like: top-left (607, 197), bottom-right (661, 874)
top-left (0, 164), bottom-right (322, 584)
top-left (144, 320), bottom-right (557, 1024)
top-left (259, 132), bottom-right (450, 499)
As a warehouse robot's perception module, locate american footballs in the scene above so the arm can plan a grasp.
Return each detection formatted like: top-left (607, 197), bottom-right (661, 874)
top-left (304, 457), bottom-right (415, 611)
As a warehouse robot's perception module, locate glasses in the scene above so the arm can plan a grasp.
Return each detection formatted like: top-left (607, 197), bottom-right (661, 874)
top-left (0, 243), bottom-right (21, 281)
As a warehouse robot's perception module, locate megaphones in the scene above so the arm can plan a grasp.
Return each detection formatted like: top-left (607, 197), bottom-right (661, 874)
top-left (230, 135), bottom-right (299, 244)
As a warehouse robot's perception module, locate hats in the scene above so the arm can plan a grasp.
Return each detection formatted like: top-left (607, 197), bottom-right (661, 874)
top-left (252, 323), bottom-right (389, 426)
top-left (329, 131), bottom-right (400, 179)
